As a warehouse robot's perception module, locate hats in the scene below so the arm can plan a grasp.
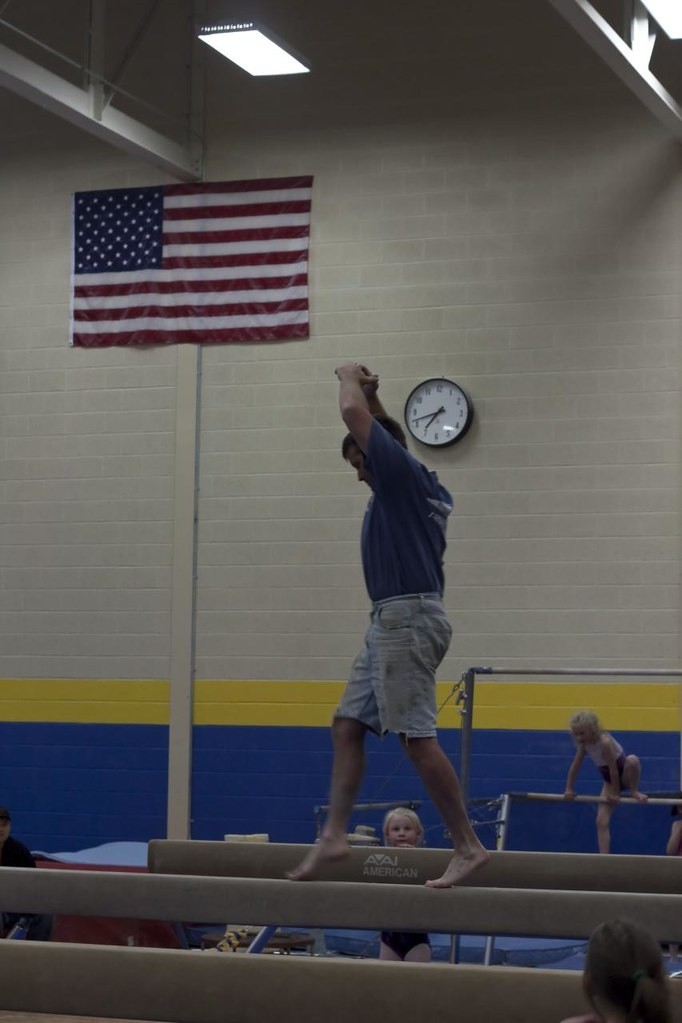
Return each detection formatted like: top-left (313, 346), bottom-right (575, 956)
top-left (0, 806), bottom-right (11, 821)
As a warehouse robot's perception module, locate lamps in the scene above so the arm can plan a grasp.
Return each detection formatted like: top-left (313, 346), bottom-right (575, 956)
top-left (195, 15), bottom-right (311, 78)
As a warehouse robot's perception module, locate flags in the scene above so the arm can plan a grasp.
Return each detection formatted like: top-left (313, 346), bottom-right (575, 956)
top-left (72, 175), bottom-right (312, 347)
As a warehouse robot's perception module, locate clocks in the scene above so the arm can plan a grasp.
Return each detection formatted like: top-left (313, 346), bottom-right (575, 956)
top-left (403, 376), bottom-right (474, 449)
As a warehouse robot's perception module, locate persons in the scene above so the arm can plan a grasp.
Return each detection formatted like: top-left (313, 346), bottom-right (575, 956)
top-left (285, 363), bottom-right (491, 888)
top-left (564, 711), bottom-right (648, 856)
top-left (0, 806), bottom-right (42, 941)
top-left (378, 808), bottom-right (431, 963)
top-left (666, 790), bottom-right (682, 960)
top-left (578, 920), bottom-right (682, 1023)
